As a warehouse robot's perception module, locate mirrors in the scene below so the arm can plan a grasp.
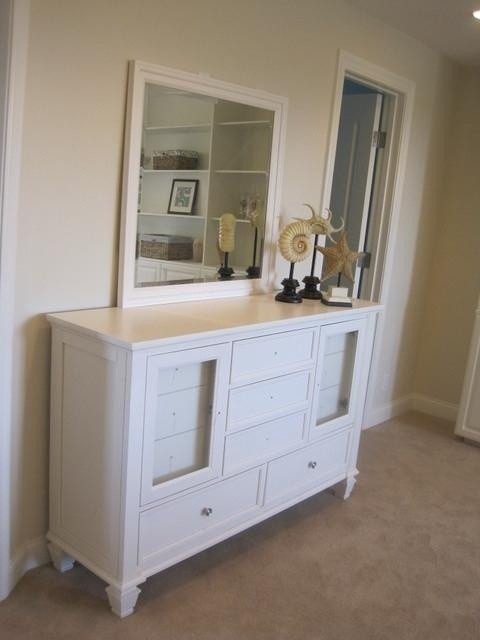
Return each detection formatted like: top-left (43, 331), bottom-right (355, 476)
top-left (117, 59), bottom-right (290, 310)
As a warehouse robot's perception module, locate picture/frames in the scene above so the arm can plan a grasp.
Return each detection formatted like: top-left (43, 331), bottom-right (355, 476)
top-left (168, 176), bottom-right (198, 215)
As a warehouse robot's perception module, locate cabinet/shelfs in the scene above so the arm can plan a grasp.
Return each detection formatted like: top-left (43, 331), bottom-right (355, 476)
top-left (46, 290), bottom-right (389, 620)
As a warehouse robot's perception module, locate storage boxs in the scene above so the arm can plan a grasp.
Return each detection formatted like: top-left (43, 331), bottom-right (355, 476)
top-left (139, 232), bottom-right (194, 262)
top-left (152, 148), bottom-right (201, 171)
